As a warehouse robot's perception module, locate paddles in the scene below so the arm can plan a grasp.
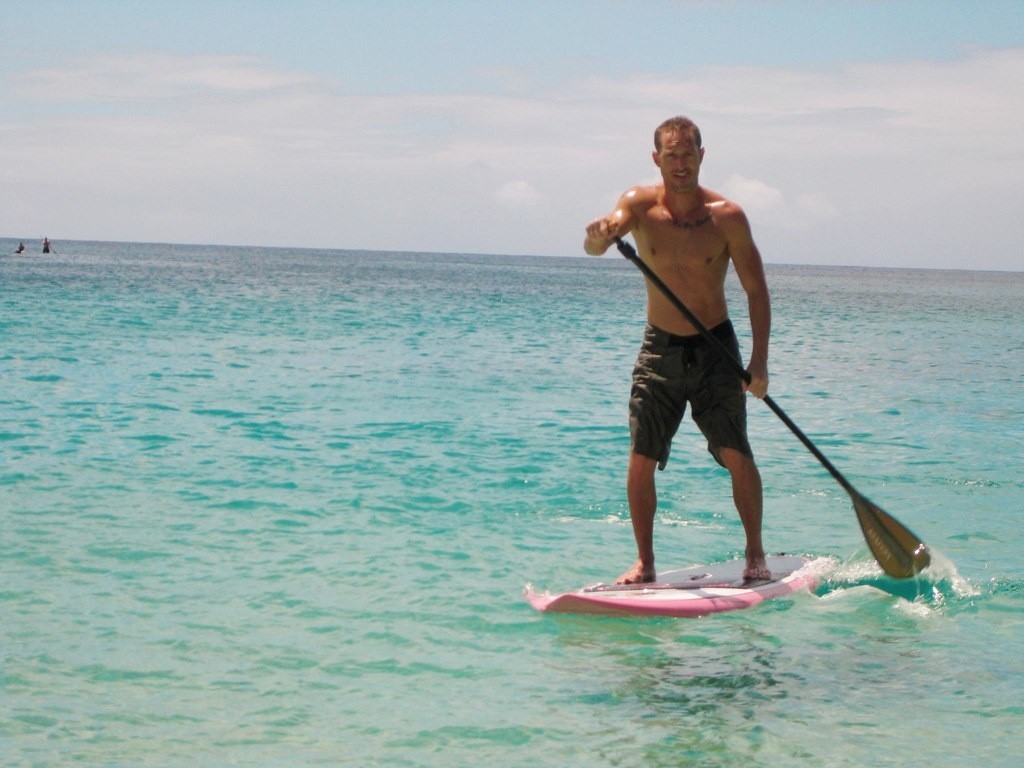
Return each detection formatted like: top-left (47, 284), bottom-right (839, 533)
top-left (611, 232), bottom-right (933, 580)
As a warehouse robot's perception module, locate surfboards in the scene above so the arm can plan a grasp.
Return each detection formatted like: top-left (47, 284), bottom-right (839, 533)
top-left (522, 554), bottom-right (820, 619)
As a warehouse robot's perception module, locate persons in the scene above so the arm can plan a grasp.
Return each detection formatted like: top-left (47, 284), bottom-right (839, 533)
top-left (43, 237), bottom-right (51, 253)
top-left (18, 242), bottom-right (25, 251)
top-left (583, 116), bottom-right (772, 585)
